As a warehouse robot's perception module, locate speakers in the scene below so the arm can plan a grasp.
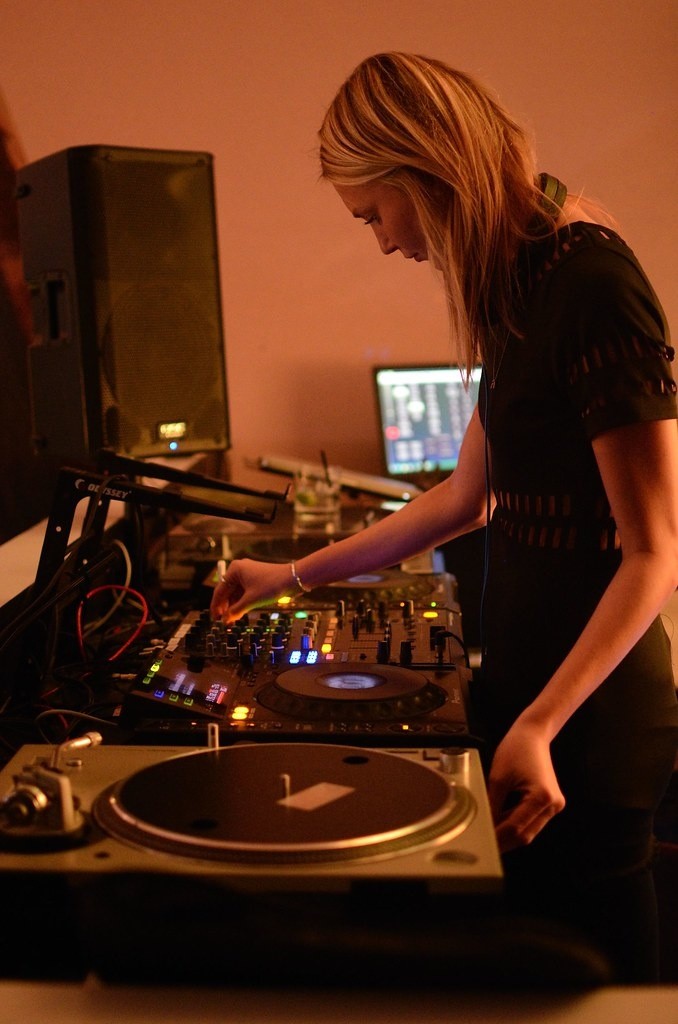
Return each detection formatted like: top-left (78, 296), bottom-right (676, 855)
top-left (13, 144), bottom-right (230, 461)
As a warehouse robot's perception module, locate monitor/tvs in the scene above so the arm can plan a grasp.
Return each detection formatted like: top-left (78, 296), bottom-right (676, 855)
top-left (372, 363), bottom-right (488, 482)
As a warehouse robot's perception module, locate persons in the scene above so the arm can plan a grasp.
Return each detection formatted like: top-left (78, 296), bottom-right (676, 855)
top-left (210, 51), bottom-right (678, 982)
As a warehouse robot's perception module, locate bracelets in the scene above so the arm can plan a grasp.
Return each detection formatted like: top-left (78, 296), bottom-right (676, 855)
top-left (289, 560), bottom-right (312, 593)
